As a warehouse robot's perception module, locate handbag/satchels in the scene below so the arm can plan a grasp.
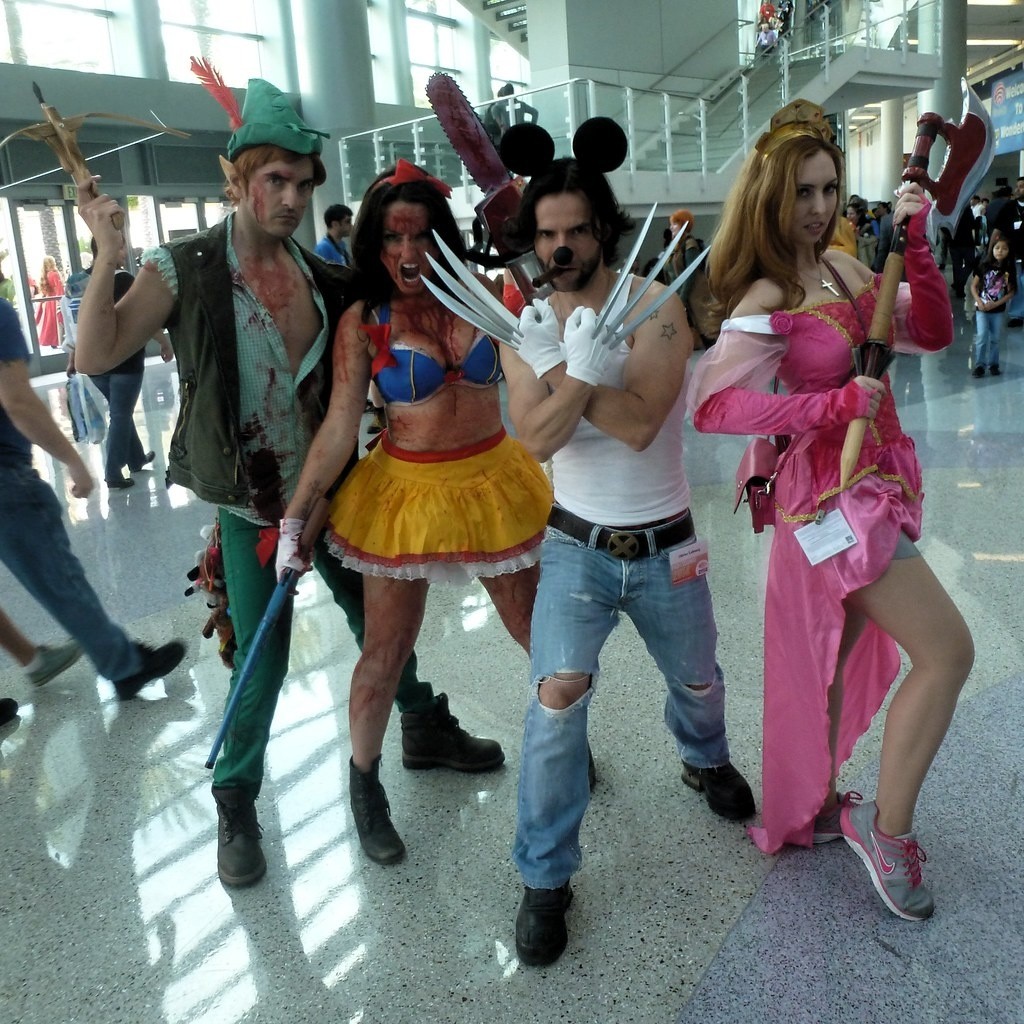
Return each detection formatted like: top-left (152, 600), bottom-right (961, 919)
top-left (65, 373), bottom-right (110, 445)
top-left (733, 438), bottom-right (779, 534)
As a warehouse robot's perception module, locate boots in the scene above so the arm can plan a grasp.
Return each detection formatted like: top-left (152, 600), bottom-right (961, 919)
top-left (349, 754), bottom-right (406, 866)
top-left (586, 742), bottom-right (596, 790)
top-left (211, 780), bottom-right (267, 886)
top-left (401, 692), bottom-right (505, 773)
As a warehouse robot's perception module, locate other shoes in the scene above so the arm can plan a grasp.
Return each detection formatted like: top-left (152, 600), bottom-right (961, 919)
top-left (0, 698), bottom-right (18, 725)
top-left (951, 281), bottom-right (966, 299)
top-left (971, 367), bottom-right (984, 377)
top-left (1008, 316), bottom-right (1024, 328)
top-left (988, 364), bottom-right (1002, 376)
top-left (113, 641), bottom-right (185, 700)
top-left (108, 474), bottom-right (134, 488)
top-left (129, 451), bottom-right (155, 472)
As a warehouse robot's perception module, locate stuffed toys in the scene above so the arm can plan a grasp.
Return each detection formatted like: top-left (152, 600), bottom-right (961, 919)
top-left (185, 548), bottom-right (237, 668)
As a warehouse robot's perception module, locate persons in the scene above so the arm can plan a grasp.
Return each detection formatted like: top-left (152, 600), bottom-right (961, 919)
top-left (969, 236), bottom-right (1018, 377)
top-left (754, 0), bottom-right (794, 58)
top-left (495, 119), bottom-right (757, 966)
top-left (313, 204), bottom-right (387, 426)
top-left (273, 162), bottom-right (597, 865)
top-left (644, 209), bottom-right (709, 350)
top-left (0, 228), bottom-right (188, 730)
top-left (689, 96), bottom-right (976, 918)
top-left (846, 175), bottom-right (1024, 327)
top-left (75, 76), bottom-right (506, 890)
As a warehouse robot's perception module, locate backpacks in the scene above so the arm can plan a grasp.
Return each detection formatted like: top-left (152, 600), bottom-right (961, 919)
top-left (65, 269), bottom-right (134, 346)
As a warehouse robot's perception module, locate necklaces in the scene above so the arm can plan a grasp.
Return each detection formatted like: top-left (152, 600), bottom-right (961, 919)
top-left (557, 270), bottom-right (611, 326)
top-left (797, 266), bottom-right (840, 298)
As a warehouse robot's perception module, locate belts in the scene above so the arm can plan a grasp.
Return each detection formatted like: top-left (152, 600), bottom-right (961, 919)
top-left (546, 506), bottom-right (694, 559)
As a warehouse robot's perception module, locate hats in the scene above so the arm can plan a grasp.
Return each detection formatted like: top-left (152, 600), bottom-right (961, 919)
top-left (187, 55), bottom-right (330, 163)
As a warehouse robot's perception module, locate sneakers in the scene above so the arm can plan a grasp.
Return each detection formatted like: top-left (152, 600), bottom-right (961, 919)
top-left (516, 877), bottom-right (574, 966)
top-left (25, 637), bottom-right (82, 687)
top-left (839, 800), bottom-right (936, 920)
top-left (811, 790), bottom-right (863, 843)
top-left (680, 760), bottom-right (757, 821)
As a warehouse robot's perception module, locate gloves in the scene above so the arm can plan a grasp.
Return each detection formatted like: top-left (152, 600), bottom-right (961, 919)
top-left (274, 518), bottom-right (316, 596)
top-left (511, 298), bottom-right (565, 380)
top-left (563, 305), bottom-right (620, 386)
